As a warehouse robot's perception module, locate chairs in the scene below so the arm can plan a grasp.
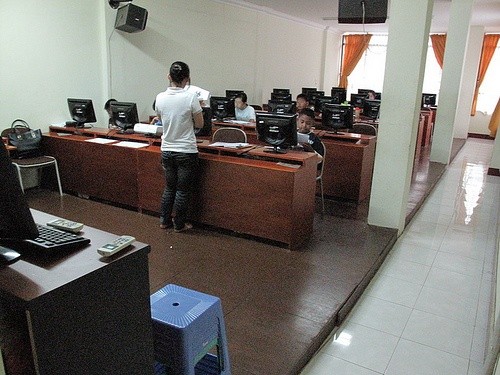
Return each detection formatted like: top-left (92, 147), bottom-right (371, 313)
top-left (1, 128), bottom-right (63, 197)
top-left (211, 127), bottom-right (246, 144)
top-left (314, 138), bottom-right (326, 216)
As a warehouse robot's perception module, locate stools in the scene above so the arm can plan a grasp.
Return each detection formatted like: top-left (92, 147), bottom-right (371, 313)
top-left (151, 284), bottom-right (232, 375)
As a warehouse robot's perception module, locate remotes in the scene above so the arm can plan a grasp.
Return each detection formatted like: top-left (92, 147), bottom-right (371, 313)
top-left (46, 219), bottom-right (84, 234)
top-left (97, 235), bottom-right (136, 256)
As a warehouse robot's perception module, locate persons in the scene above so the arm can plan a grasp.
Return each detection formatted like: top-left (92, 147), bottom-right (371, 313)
top-left (104, 99), bottom-right (117, 129)
top-left (150, 100), bottom-right (162, 126)
top-left (235, 92), bottom-right (256, 122)
top-left (294, 93), bottom-right (314, 120)
top-left (155, 61), bottom-right (204, 232)
top-left (368, 90), bottom-right (376, 99)
top-left (297, 108), bottom-right (324, 167)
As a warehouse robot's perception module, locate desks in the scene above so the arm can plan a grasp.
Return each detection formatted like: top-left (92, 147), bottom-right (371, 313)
top-left (211, 120), bottom-right (376, 206)
top-left (40, 125), bottom-right (318, 250)
top-left (0, 209), bottom-right (155, 375)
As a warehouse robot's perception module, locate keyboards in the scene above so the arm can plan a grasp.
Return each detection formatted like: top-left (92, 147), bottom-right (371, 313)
top-left (20, 222), bottom-right (90, 258)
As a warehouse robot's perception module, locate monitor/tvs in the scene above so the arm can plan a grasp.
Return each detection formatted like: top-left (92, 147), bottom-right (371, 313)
top-left (192, 86), bottom-right (381, 138)
top-left (67, 98), bottom-right (97, 128)
top-left (0, 163), bottom-right (38, 268)
top-left (422, 93), bottom-right (436, 109)
top-left (255, 112), bottom-right (297, 154)
top-left (109, 101), bottom-right (140, 135)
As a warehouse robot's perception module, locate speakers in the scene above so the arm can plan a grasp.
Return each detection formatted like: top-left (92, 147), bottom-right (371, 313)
top-left (114, 3), bottom-right (148, 33)
top-left (338, 0), bottom-right (388, 24)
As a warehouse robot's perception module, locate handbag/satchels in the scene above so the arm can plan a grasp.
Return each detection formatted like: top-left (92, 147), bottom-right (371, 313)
top-left (7, 120), bottom-right (45, 160)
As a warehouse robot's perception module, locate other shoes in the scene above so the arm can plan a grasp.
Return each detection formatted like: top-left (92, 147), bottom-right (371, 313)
top-left (160, 218), bottom-right (175, 229)
top-left (174, 222), bottom-right (194, 233)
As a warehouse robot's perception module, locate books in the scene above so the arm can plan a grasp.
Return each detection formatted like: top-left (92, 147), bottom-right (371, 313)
top-left (208, 142), bottom-right (252, 149)
top-left (297, 132), bottom-right (310, 143)
top-left (184, 84), bottom-right (209, 101)
